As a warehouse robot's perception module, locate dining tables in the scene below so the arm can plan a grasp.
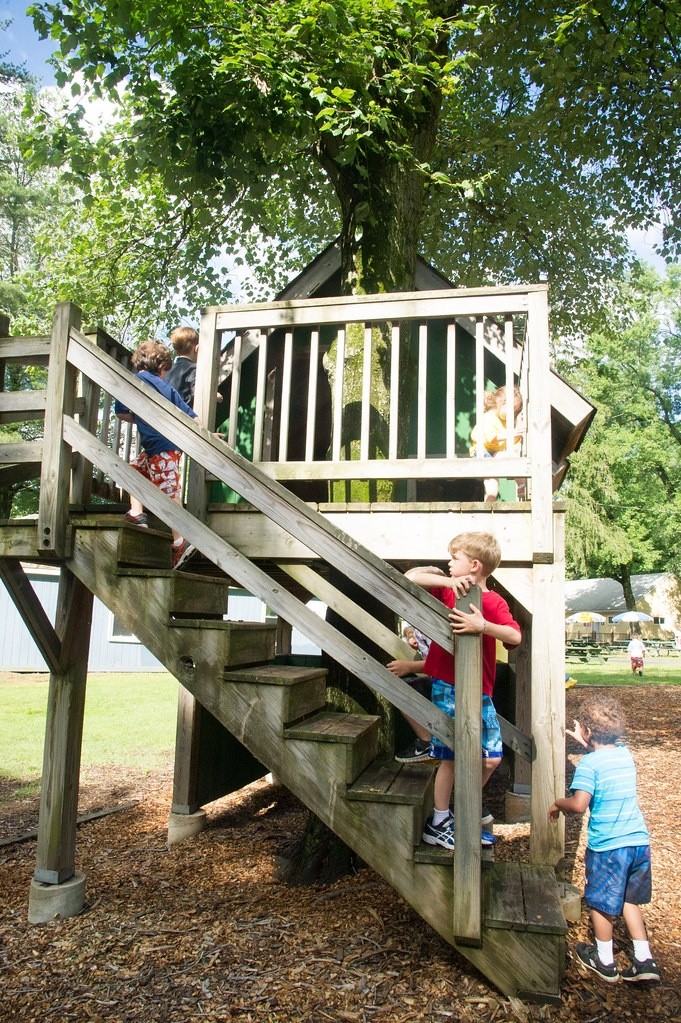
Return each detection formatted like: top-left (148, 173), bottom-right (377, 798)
top-left (564, 638), bottom-right (679, 665)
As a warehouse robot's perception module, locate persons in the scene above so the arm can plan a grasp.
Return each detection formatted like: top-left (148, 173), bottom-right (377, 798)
top-left (113, 339), bottom-right (197, 569)
top-left (403, 531), bottom-right (522, 850)
top-left (546, 694), bottom-right (662, 983)
top-left (384, 565), bottom-right (450, 763)
top-left (627, 633), bottom-right (646, 676)
top-left (468, 386), bottom-right (529, 504)
top-left (163, 327), bottom-right (224, 413)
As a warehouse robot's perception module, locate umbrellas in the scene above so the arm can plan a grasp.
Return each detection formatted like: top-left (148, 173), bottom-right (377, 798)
top-left (566, 612), bottom-right (606, 643)
top-left (612, 610), bottom-right (654, 633)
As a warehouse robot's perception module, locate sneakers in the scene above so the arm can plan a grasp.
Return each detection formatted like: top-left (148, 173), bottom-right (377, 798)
top-left (394, 736), bottom-right (436, 761)
top-left (619, 956), bottom-right (660, 984)
top-left (575, 941), bottom-right (619, 982)
top-left (422, 816), bottom-right (455, 848)
top-left (169, 537), bottom-right (196, 569)
top-left (449, 803), bottom-right (494, 825)
top-left (449, 819), bottom-right (495, 845)
top-left (115, 509), bottom-right (147, 527)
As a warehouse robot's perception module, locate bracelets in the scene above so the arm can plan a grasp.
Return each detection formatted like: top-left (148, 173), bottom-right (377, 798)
top-left (481, 619), bottom-right (487, 635)
